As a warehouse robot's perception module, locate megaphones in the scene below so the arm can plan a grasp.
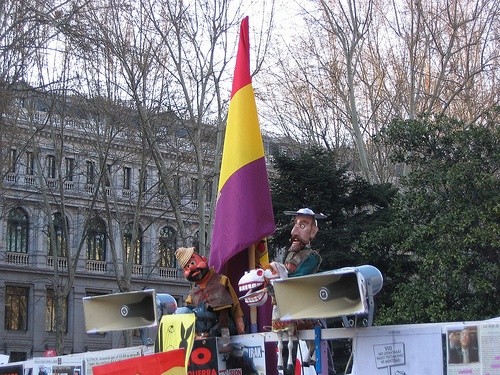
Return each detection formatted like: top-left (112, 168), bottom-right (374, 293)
top-left (272, 264), bottom-right (384, 322)
top-left (82, 287), bottom-right (178, 336)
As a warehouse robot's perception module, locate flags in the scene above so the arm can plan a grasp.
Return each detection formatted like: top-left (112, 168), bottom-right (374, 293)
top-left (208, 17), bottom-right (279, 375)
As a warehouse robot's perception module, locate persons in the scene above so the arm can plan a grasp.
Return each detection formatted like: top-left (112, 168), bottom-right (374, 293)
top-left (175, 247), bottom-right (245, 336)
top-left (282, 208), bottom-right (328, 278)
top-left (449, 329), bottom-right (478, 363)
